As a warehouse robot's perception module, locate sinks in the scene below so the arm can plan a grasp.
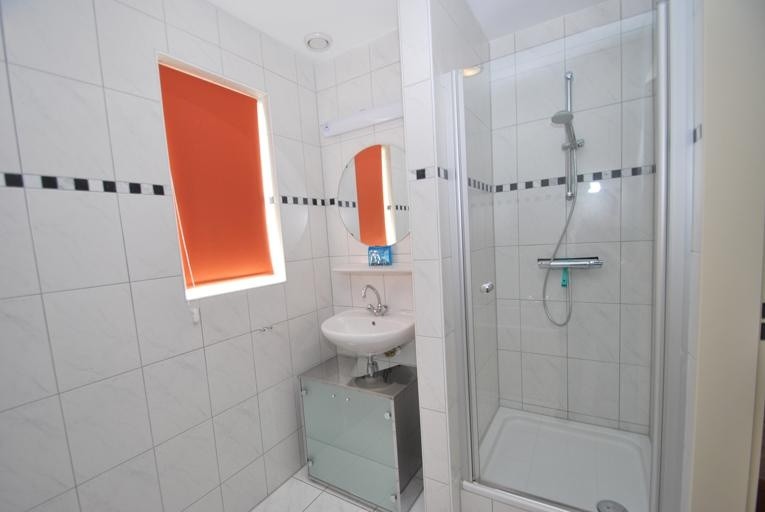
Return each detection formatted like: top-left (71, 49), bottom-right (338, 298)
top-left (321, 308), bottom-right (415, 357)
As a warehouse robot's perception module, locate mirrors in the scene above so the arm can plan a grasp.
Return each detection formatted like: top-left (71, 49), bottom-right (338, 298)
top-left (337, 144), bottom-right (409, 247)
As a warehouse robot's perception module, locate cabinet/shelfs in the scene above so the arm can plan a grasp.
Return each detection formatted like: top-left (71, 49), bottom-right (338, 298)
top-left (298, 355), bottom-right (423, 512)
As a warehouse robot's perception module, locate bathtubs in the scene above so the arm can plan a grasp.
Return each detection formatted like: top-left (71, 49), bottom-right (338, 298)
top-left (475, 406), bottom-right (651, 512)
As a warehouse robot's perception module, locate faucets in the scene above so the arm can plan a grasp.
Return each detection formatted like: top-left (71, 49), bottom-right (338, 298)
top-left (362, 285), bottom-right (388, 316)
